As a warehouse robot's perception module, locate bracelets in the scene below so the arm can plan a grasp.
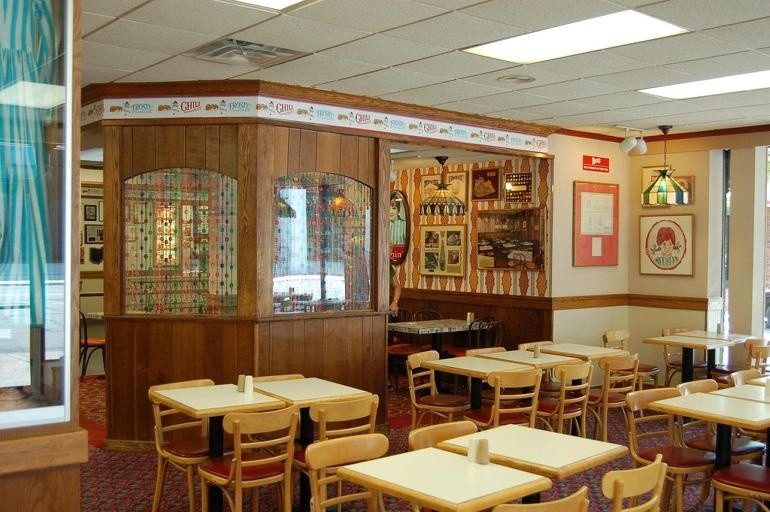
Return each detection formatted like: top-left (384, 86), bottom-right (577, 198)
top-left (391, 299), bottom-right (398, 302)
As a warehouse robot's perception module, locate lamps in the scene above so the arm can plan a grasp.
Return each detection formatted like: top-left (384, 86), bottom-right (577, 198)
top-left (418, 156), bottom-right (466, 217)
top-left (326, 188), bottom-right (356, 213)
top-left (617, 126), bottom-right (649, 157)
top-left (278, 189), bottom-right (296, 219)
top-left (640, 124), bottom-right (691, 207)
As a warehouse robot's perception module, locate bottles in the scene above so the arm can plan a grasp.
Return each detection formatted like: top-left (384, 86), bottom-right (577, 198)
top-left (716, 323), bottom-right (722, 335)
top-left (243, 375), bottom-right (254, 394)
top-left (272, 292), bottom-right (357, 313)
top-left (476, 439), bottom-right (490, 465)
top-left (237, 374), bottom-right (246, 392)
top-left (466, 312), bottom-right (475, 326)
top-left (467, 438), bottom-right (478, 463)
top-left (533, 343), bottom-right (541, 358)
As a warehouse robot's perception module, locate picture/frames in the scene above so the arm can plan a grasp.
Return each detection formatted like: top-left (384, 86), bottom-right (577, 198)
top-left (504, 171), bottom-right (536, 205)
top-left (670, 175), bottom-right (696, 204)
top-left (475, 205), bottom-right (548, 273)
top-left (640, 164), bottom-right (672, 210)
top-left (638, 213), bottom-right (695, 278)
top-left (390, 189), bottom-right (412, 266)
top-left (469, 165), bottom-right (502, 202)
top-left (572, 180), bottom-right (619, 267)
top-left (419, 170), bottom-right (470, 212)
top-left (419, 223), bottom-right (468, 278)
top-left (79, 200), bottom-right (148, 264)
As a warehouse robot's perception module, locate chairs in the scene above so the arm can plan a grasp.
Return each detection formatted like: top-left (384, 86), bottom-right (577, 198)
top-left (478, 238), bottom-right (545, 269)
top-left (78, 309), bottom-right (107, 384)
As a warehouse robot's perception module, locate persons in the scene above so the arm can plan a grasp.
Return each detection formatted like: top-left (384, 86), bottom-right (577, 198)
top-left (388, 196), bottom-right (405, 245)
top-left (387, 261), bottom-right (403, 393)
